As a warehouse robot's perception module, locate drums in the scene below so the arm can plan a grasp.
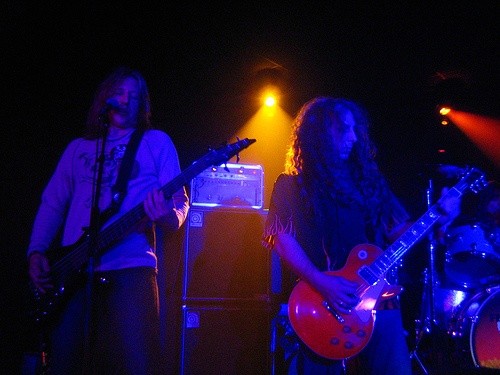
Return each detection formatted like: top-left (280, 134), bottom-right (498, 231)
top-left (414, 265), bottom-right (478, 363)
top-left (441, 223), bottom-right (500, 290)
top-left (450, 284), bottom-right (500, 370)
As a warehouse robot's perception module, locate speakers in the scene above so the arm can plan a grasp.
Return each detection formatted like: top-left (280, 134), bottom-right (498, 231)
top-left (165, 209), bottom-right (273, 302)
top-left (153, 306), bottom-right (275, 375)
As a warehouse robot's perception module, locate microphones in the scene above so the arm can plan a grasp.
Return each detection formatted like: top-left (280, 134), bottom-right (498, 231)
top-left (99, 97), bottom-right (118, 118)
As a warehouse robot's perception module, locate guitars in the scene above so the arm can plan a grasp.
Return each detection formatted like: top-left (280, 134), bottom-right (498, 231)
top-left (287, 163), bottom-right (488, 360)
top-left (0, 137), bottom-right (254, 347)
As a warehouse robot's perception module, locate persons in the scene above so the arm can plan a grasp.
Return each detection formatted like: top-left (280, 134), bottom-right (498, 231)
top-left (28, 68), bottom-right (188, 375)
top-left (264, 96), bottom-right (462, 375)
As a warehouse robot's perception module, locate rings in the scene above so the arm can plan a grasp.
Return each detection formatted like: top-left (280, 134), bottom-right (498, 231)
top-left (339, 301), bottom-right (344, 305)
